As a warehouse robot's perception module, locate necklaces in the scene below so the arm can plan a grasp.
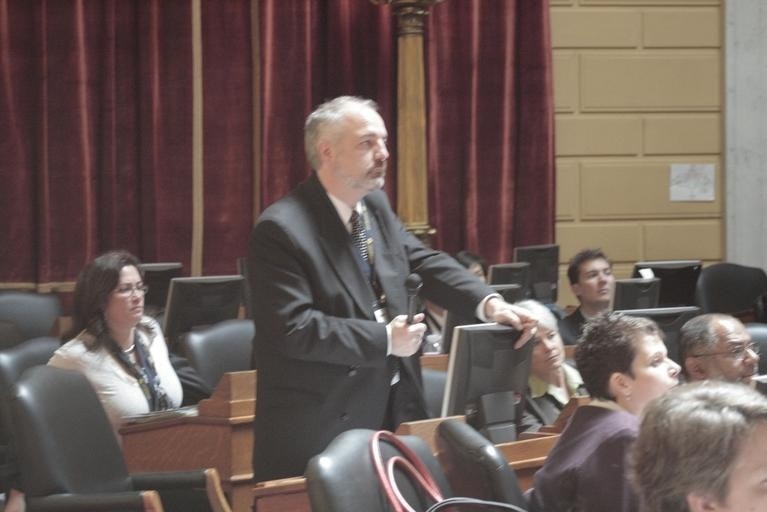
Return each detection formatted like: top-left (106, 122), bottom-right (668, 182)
top-left (114, 341), bottom-right (141, 356)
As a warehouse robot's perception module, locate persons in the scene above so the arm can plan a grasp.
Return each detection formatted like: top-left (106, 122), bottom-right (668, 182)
top-left (45, 248), bottom-right (184, 442)
top-left (560, 250), bottom-right (631, 348)
top-left (527, 314), bottom-right (683, 512)
top-left (670, 313), bottom-right (760, 391)
top-left (418, 294), bottom-right (446, 337)
top-left (245, 97), bottom-right (540, 485)
top-left (624, 380), bottom-right (767, 512)
top-left (449, 248), bottom-right (492, 292)
top-left (501, 298), bottom-right (586, 442)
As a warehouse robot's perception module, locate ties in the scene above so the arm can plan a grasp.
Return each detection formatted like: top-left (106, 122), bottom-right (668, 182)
top-left (351, 212), bottom-right (368, 261)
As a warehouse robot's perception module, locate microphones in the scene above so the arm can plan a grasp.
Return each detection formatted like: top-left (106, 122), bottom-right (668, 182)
top-left (404, 273), bottom-right (424, 325)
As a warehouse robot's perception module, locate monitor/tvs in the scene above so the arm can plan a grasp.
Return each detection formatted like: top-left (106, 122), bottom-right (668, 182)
top-left (614, 306), bottom-right (705, 375)
top-left (440, 322), bottom-right (536, 445)
top-left (490, 262), bottom-right (533, 300)
top-left (139, 261), bottom-right (185, 309)
top-left (613, 278), bottom-right (662, 310)
top-left (631, 260), bottom-right (703, 309)
top-left (441, 284), bottom-right (523, 355)
top-left (514, 245), bottom-right (559, 306)
top-left (163, 274), bottom-right (246, 339)
